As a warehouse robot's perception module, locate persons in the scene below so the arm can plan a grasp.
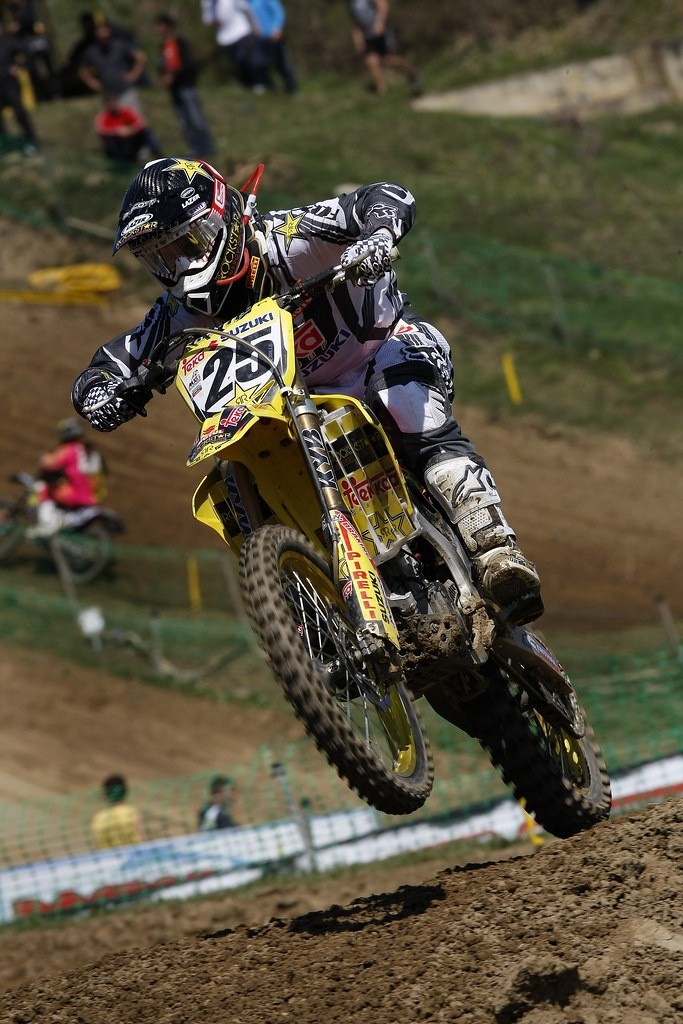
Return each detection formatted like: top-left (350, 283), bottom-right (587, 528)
top-left (26, 417), bottom-right (114, 539)
top-left (71, 155), bottom-right (545, 629)
top-left (0, 0), bottom-right (424, 178)
top-left (89, 773), bottom-right (146, 847)
top-left (196, 775), bottom-right (240, 832)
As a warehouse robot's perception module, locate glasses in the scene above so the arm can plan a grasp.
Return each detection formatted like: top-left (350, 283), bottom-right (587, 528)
top-left (130, 220), bottom-right (217, 279)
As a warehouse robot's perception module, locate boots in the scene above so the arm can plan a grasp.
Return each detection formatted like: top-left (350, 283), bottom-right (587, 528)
top-left (421, 450), bottom-right (544, 627)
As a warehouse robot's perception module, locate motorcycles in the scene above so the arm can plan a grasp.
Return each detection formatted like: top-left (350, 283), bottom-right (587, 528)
top-left (0, 470), bottom-right (127, 590)
top-left (107, 237), bottom-right (615, 840)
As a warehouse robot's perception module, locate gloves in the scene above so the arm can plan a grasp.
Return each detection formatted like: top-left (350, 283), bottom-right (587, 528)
top-left (81, 379), bottom-right (136, 432)
top-left (340, 232), bottom-right (394, 290)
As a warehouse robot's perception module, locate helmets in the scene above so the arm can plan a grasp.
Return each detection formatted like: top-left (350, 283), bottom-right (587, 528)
top-left (57, 418), bottom-right (84, 443)
top-left (112, 156), bottom-right (244, 316)
